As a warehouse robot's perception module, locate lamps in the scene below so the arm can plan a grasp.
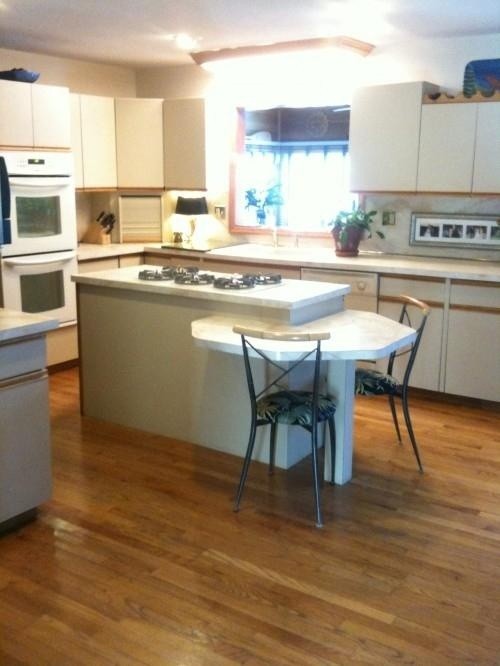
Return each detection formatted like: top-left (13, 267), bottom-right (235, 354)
top-left (176, 196), bottom-right (208, 241)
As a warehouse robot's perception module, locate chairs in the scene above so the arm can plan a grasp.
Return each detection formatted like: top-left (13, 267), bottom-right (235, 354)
top-left (354, 295), bottom-right (431, 474)
top-left (232, 324), bottom-right (339, 529)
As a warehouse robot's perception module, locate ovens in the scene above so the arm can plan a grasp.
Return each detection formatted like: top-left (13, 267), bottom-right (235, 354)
top-left (0, 149), bottom-right (79, 331)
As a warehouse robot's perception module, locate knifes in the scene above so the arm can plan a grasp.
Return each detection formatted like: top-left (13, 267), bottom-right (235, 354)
top-left (96, 211), bottom-right (116, 235)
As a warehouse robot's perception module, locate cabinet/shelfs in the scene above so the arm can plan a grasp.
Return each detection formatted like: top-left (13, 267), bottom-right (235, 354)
top-left (377, 274), bottom-right (500, 403)
top-left (300, 257), bottom-right (377, 313)
top-left (115, 97), bottom-right (164, 189)
top-left (162, 98), bottom-right (208, 191)
top-left (0, 79), bottom-right (72, 149)
top-left (417, 101), bottom-right (500, 195)
top-left (345, 81), bottom-right (438, 193)
top-left (78, 256), bottom-right (300, 281)
top-left (72, 92), bottom-right (117, 189)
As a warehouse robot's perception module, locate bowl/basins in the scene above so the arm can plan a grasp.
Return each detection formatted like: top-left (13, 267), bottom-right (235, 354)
top-left (0, 70), bottom-right (41, 83)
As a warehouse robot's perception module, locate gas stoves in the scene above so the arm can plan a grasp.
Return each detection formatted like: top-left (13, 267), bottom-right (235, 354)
top-left (137, 262), bottom-right (287, 294)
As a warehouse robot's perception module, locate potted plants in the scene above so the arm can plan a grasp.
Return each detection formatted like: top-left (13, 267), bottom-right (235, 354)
top-left (243, 183), bottom-right (281, 225)
top-left (329, 200), bottom-right (385, 257)
